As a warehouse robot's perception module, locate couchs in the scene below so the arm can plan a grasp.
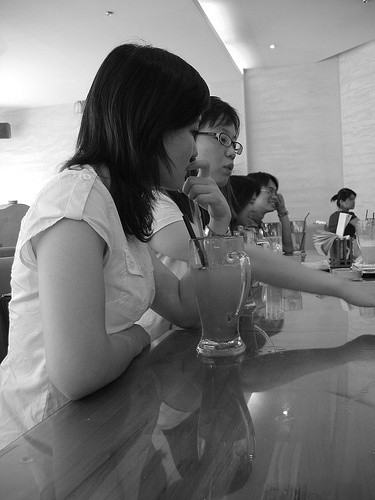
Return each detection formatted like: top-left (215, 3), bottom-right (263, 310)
top-left (0, 200), bottom-right (30, 298)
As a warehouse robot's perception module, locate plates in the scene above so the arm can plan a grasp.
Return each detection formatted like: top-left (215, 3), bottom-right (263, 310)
top-left (351, 260), bottom-right (375, 273)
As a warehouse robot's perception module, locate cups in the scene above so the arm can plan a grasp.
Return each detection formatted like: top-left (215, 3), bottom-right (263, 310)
top-left (290, 220), bottom-right (308, 252)
top-left (234, 221), bottom-right (282, 307)
top-left (356, 220), bottom-right (375, 262)
top-left (189, 235), bottom-right (252, 357)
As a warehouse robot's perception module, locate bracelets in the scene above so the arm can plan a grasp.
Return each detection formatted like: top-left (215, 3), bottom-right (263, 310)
top-left (278, 211), bottom-right (289, 217)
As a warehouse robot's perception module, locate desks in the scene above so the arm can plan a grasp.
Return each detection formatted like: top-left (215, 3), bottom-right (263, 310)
top-left (0, 251), bottom-right (375, 500)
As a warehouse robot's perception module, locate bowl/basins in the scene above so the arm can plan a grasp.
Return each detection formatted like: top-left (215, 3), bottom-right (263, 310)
top-left (332, 268), bottom-right (362, 279)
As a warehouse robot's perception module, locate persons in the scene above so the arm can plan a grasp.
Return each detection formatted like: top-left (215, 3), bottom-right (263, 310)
top-left (17, 330), bottom-right (374, 499)
top-left (0, 280), bottom-right (375, 500)
top-left (244, 170), bottom-right (293, 256)
top-left (327, 187), bottom-right (359, 240)
top-left (138, 96), bottom-right (375, 342)
top-left (217, 174), bottom-right (260, 242)
top-left (1, 44), bottom-right (212, 451)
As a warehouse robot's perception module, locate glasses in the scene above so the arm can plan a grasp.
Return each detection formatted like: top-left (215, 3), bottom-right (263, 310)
top-left (197, 132), bottom-right (243, 155)
top-left (261, 189), bottom-right (277, 196)
